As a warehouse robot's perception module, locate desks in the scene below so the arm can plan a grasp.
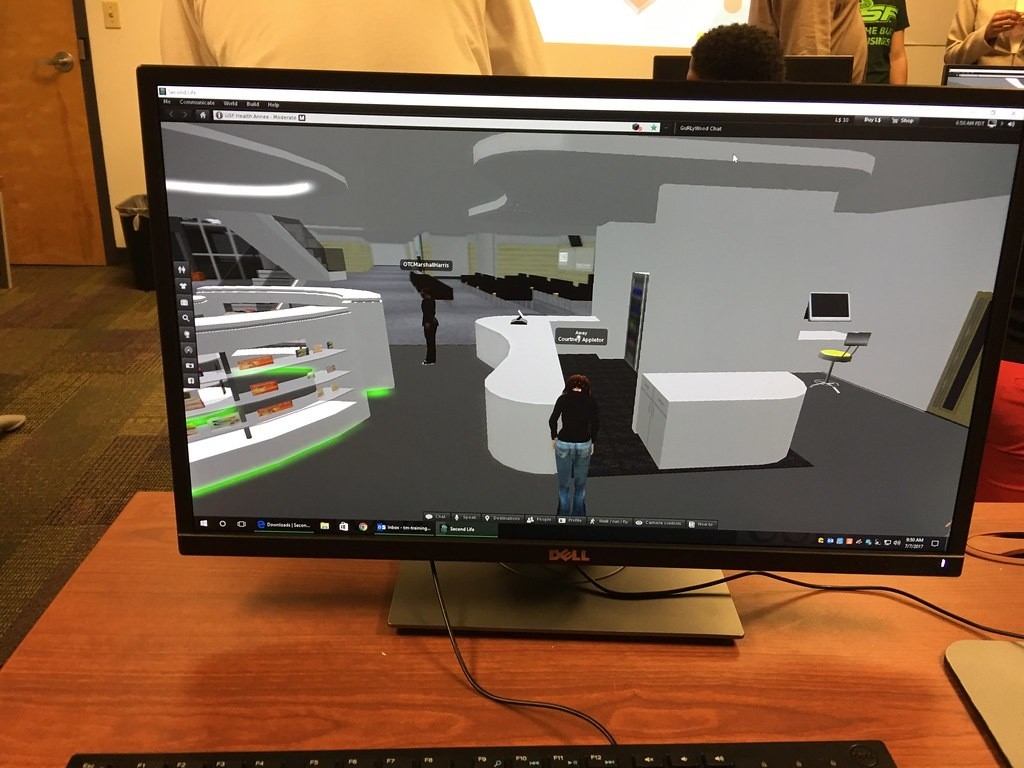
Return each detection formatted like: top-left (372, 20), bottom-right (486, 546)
top-left (0, 492), bottom-right (1024, 768)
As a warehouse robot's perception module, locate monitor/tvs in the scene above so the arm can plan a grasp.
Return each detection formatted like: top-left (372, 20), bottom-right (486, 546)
top-left (941, 65), bottom-right (1024, 89)
top-left (654, 56), bottom-right (853, 83)
top-left (137, 64), bottom-right (1024, 638)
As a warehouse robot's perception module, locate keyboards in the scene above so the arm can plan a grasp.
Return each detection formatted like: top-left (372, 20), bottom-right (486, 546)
top-left (66, 739), bottom-right (898, 768)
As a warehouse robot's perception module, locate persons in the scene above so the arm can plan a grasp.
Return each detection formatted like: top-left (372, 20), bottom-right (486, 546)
top-left (421, 287), bottom-right (439, 366)
top-left (687, 0), bottom-right (1024, 83)
top-left (548, 373), bottom-right (601, 517)
top-left (159, 1), bottom-right (547, 78)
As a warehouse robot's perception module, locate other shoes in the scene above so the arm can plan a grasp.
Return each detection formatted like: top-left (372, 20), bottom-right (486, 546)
top-left (0, 415), bottom-right (27, 432)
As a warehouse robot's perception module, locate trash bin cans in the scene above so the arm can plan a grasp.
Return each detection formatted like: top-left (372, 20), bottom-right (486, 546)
top-left (116, 195), bottom-right (156, 291)
